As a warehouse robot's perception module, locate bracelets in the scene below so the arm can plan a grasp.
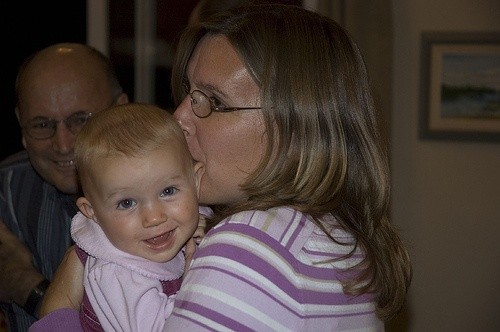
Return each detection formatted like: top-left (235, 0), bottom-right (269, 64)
top-left (23, 279), bottom-right (50, 316)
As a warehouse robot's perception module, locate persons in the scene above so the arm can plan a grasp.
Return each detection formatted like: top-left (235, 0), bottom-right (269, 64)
top-left (69, 103), bottom-right (214, 332)
top-left (0, 42), bottom-right (130, 332)
top-left (26, 2), bottom-right (389, 332)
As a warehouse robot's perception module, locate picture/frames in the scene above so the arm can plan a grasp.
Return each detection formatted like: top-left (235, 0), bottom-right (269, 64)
top-left (417, 30), bottom-right (500, 144)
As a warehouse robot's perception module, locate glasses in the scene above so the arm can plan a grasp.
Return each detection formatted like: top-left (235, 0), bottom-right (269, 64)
top-left (182, 78), bottom-right (277, 119)
top-left (19, 91), bottom-right (125, 140)
